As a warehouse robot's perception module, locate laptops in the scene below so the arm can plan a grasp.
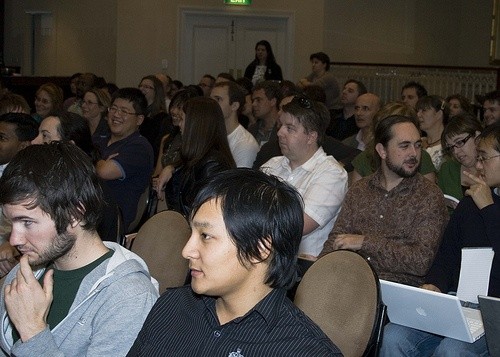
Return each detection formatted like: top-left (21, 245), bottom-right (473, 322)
top-left (379, 278), bottom-right (487, 343)
top-left (477, 295), bottom-right (500, 357)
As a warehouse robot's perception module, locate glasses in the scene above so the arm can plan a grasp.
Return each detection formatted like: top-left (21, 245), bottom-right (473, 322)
top-left (81, 100), bottom-right (98, 105)
top-left (138, 84), bottom-right (155, 89)
top-left (198, 83), bottom-right (211, 87)
top-left (446, 134), bottom-right (473, 152)
top-left (108, 107), bottom-right (136, 116)
top-left (475, 155), bottom-right (500, 163)
top-left (36, 96), bottom-right (53, 104)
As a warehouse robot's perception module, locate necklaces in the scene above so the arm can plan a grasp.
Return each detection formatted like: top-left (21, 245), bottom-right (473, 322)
top-left (426, 138), bottom-right (441, 149)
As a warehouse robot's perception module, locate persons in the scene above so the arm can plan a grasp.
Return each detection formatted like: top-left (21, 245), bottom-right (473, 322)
top-left (0, 40), bottom-right (500, 357)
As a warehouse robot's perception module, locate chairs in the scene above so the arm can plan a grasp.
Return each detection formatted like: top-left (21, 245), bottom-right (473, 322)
top-left (292, 248), bottom-right (388, 357)
top-left (130, 210), bottom-right (192, 297)
